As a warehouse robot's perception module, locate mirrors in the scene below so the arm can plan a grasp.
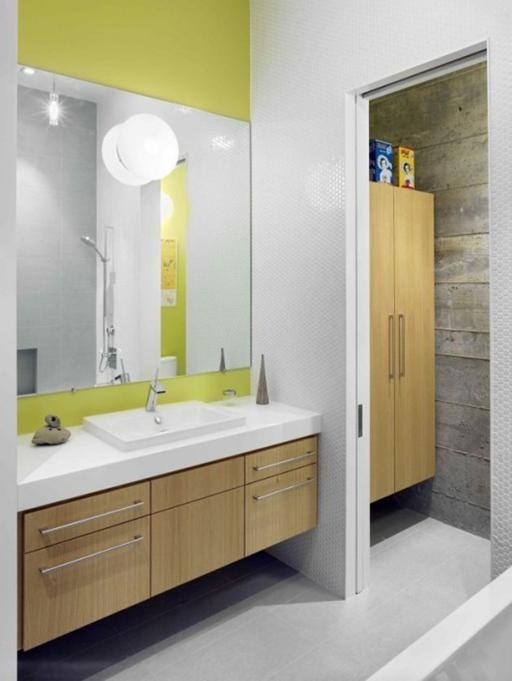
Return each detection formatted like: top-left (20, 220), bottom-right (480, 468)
top-left (17, 67), bottom-right (253, 399)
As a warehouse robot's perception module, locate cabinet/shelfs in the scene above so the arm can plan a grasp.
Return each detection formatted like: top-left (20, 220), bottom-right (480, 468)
top-left (244, 434), bottom-right (319, 559)
top-left (15, 481), bottom-right (150, 651)
top-left (150, 454), bottom-right (246, 598)
top-left (370, 179), bottom-right (436, 503)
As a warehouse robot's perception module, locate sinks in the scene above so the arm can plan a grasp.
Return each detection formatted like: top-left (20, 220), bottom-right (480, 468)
top-left (83, 401), bottom-right (246, 451)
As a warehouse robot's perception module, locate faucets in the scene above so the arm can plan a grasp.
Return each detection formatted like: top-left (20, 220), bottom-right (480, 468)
top-left (145, 369), bottom-right (166, 412)
top-left (115, 361), bottom-right (130, 383)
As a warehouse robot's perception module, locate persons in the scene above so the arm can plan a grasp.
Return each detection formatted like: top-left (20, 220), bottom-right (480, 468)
top-left (380, 158), bottom-right (392, 183)
top-left (402, 163), bottom-right (414, 191)
